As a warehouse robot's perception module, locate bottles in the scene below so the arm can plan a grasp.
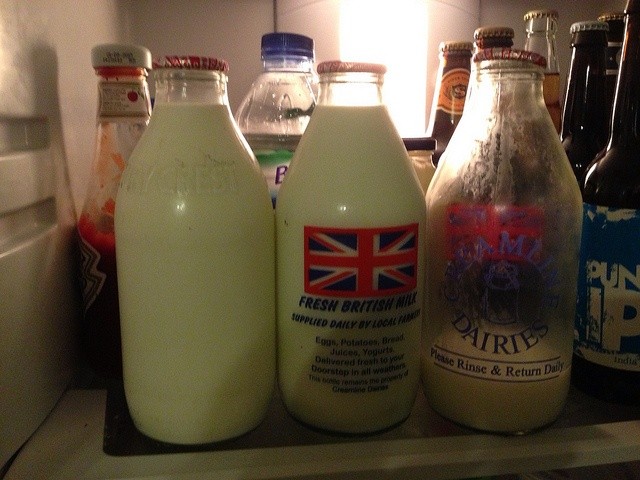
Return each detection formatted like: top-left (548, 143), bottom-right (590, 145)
top-left (520, 10), bottom-right (560, 126)
top-left (232, 32), bottom-right (315, 206)
top-left (582, 0), bottom-right (639, 419)
top-left (75, 42), bottom-right (152, 385)
top-left (427, 42), bottom-right (474, 167)
top-left (596, 13), bottom-right (626, 85)
top-left (426, 47), bottom-right (583, 437)
top-left (120, 54), bottom-right (277, 446)
top-left (278, 60), bottom-right (426, 436)
top-left (559, 20), bottom-right (608, 188)
top-left (470, 26), bottom-right (515, 50)
top-left (401, 137), bottom-right (437, 192)
